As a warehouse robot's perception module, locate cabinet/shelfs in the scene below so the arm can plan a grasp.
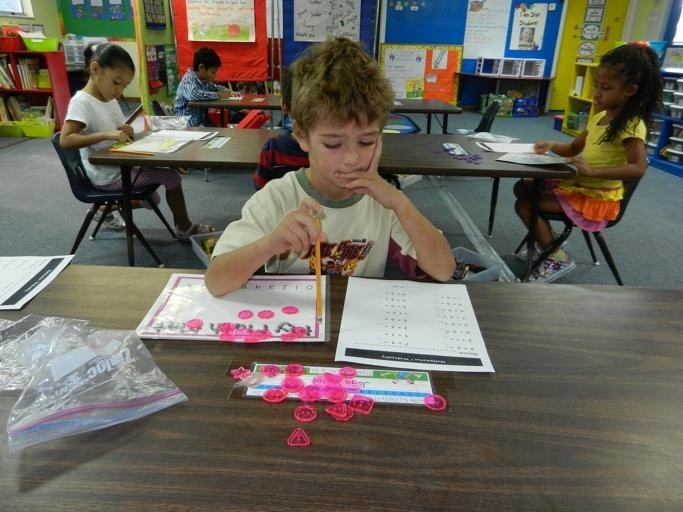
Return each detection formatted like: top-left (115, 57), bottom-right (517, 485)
top-left (0, 50), bottom-right (72, 133)
top-left (559, 61), bottom-right (683, 180)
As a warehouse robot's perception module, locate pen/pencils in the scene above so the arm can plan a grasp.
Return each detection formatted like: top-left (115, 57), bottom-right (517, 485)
top-left (110, 148), bottom-right (154, 155)
top-left (312, 210), bottom-right (323, 322)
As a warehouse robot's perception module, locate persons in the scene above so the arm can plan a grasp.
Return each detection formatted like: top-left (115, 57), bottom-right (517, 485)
top-left (204, 37), bottom-right (457, 297)
top-left (513, 41), bottom-right (664, 284)
top-left (253, 67), bottom-right (310, 191)
top-left (174, 47), bottom-right (241, 174)
top-left (59, 43), bottom-right (216, 241)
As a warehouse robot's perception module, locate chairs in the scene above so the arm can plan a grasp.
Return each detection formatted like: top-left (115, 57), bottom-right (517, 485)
top-left (149, 99), bottom-right (167, 115)
top-left (49, 129), bottom-right (178, 269)
top-left (515, 155), bottom-right (650, 286)
top-left (447, 99), bottom-right (502, 136)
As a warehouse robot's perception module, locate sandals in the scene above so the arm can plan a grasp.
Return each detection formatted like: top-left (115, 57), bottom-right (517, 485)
top-left (87, 207), bottom-right (126, 229)
top-left (176, 223), bottom-right (215, 240)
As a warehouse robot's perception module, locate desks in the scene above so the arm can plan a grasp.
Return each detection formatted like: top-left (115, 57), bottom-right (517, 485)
top-left (454, 71), bottom-right (557, 116)
top-left (1, 262), bottom-right (683, 512)
top-left (87, 125), bottom-right (579, 268)
top-left (187, 93), bottom-right (464, 134)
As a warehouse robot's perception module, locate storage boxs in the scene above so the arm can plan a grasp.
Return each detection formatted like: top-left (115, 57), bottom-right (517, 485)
top-left (22, 36), bottom-right (61, 52)
top-left (512, 95), bottom-right (541, 118)
top-left (0, 120), bottom-right (24, 138)
top-left (20, 116), bottom-right (56, 138)
top-left (0, 36), bottom-right (26, 51)
top-left (479, 93), bottom-right (514, 119)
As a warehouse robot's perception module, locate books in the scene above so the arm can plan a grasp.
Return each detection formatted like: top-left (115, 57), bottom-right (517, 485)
top-left (0, 26), bottom-right (55, 124)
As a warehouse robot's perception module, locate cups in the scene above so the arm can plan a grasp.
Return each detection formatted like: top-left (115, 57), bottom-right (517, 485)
top-left (68, 34), bottom-right (77, 44)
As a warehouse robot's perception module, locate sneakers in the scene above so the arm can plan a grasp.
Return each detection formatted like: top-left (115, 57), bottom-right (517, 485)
top-left (529, 254), bottom-right (576, 284)
top-left (521, 234), bottom-right (569, 257)
